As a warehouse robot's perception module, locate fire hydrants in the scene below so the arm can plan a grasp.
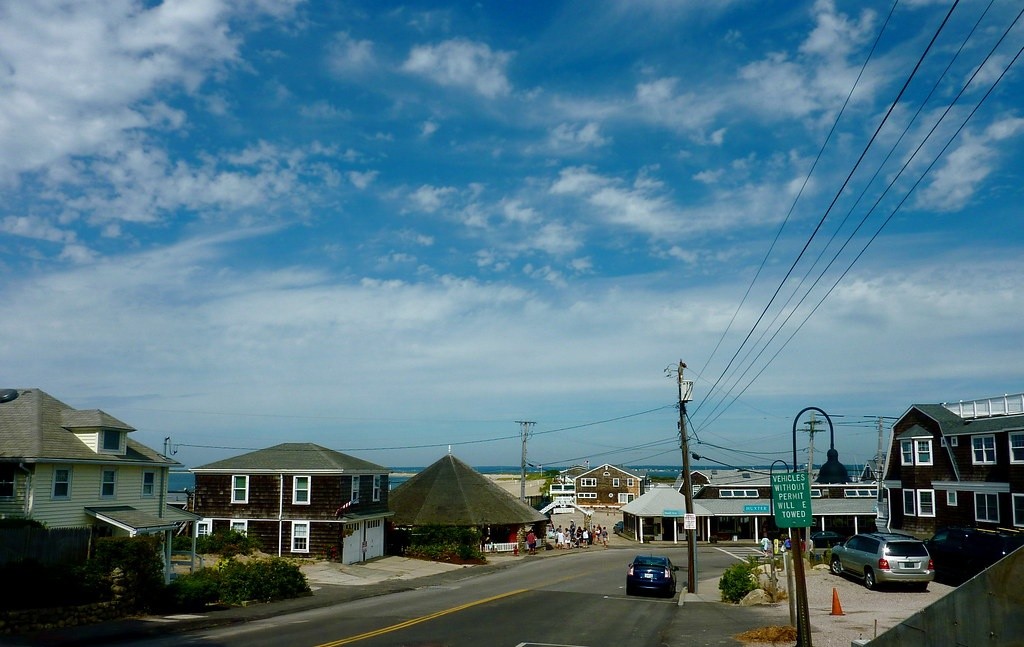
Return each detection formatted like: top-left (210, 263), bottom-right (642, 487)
top-left (512, 545), bottom-right (518, 557)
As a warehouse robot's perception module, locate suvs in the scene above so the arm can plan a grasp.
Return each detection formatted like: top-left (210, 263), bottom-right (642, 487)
top-left (830, 531), bottom-right (936, 590)
top-left (922, 527), bottom-right (1024, 587)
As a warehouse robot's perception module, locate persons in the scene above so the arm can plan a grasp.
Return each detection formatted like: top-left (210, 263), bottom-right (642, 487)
top-left (781, 541), bottom-right (786, 552)
top-left (761, 536), bottom-right (771, 551)
top-left (601, 527), bottom-right (608, 548)
top-left (613, 524), bottom-right (619, 533)
top-left (547, 519), bottom-right (602, 550)
top-left (784, 537), bottom-right (791, 551)
top-left (527, 531), bottom-right (536, 555)
top-left (809, 539), bottom-right (814, 566)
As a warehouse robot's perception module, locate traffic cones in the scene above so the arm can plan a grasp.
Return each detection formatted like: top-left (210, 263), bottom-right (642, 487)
top-left (830, 588), bottom-right (846, 615)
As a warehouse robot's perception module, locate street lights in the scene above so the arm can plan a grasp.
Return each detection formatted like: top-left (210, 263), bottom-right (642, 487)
top-left (792, 406), bottom-right (853, 647)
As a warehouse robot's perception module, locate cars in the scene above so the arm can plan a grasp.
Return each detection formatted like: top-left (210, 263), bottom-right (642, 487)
top-left (612, 521), bottom-right (624, 533)
top-left (626, 554), bottom-right (679, 598)
top-left (810, 530), bottom-right (848, 547)
